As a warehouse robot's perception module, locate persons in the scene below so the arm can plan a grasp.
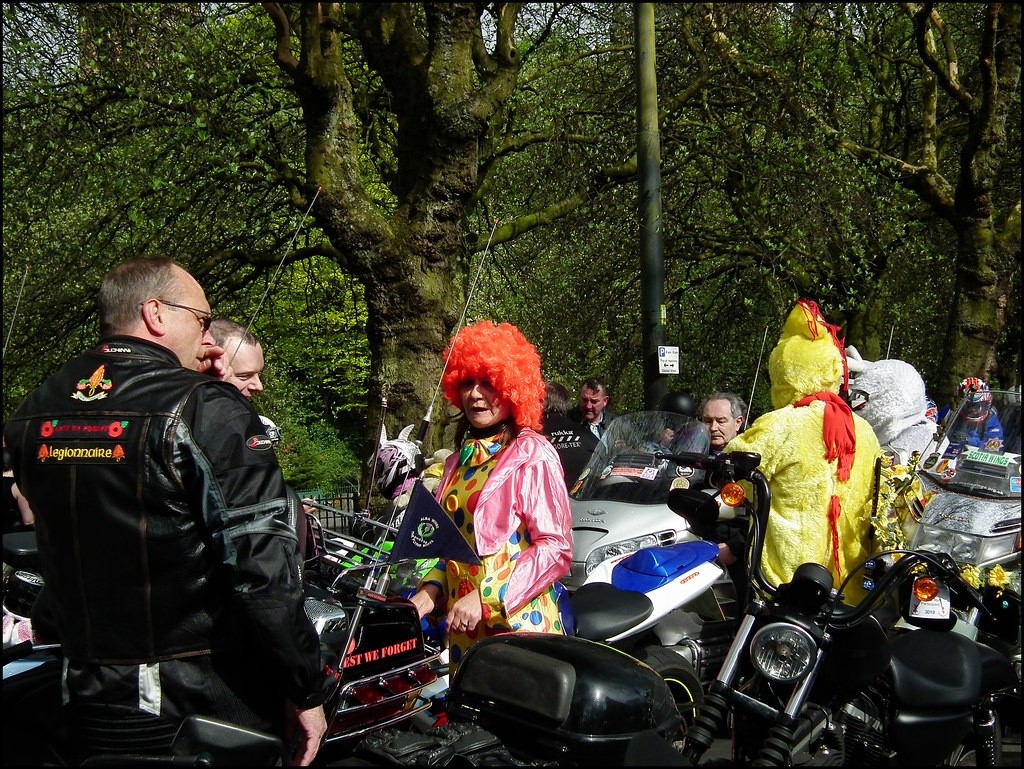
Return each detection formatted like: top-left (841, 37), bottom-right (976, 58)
top-left (0, 255), bottom-right (327, 766)
top-left (366, 298), bottom-right (1004, 725)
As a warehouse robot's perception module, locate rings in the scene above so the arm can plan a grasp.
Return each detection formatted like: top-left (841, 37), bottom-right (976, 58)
top-left (460, 622), bottom-right (468, 627)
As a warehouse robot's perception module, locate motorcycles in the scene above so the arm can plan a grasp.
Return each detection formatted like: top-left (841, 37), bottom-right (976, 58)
top-left (1, 393), bottom-right (1024, 768)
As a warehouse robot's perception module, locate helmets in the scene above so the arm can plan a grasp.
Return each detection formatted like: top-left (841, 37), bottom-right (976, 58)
top-left (955, 376), bottom-right (993, 425)
top-left (659, 392), bottom-right (696, 417)
top-left (368, 440), bottom-right (422, 498)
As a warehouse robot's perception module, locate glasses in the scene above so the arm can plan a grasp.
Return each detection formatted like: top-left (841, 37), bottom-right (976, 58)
top-left (140, 297), bottom-right (212, 331)
